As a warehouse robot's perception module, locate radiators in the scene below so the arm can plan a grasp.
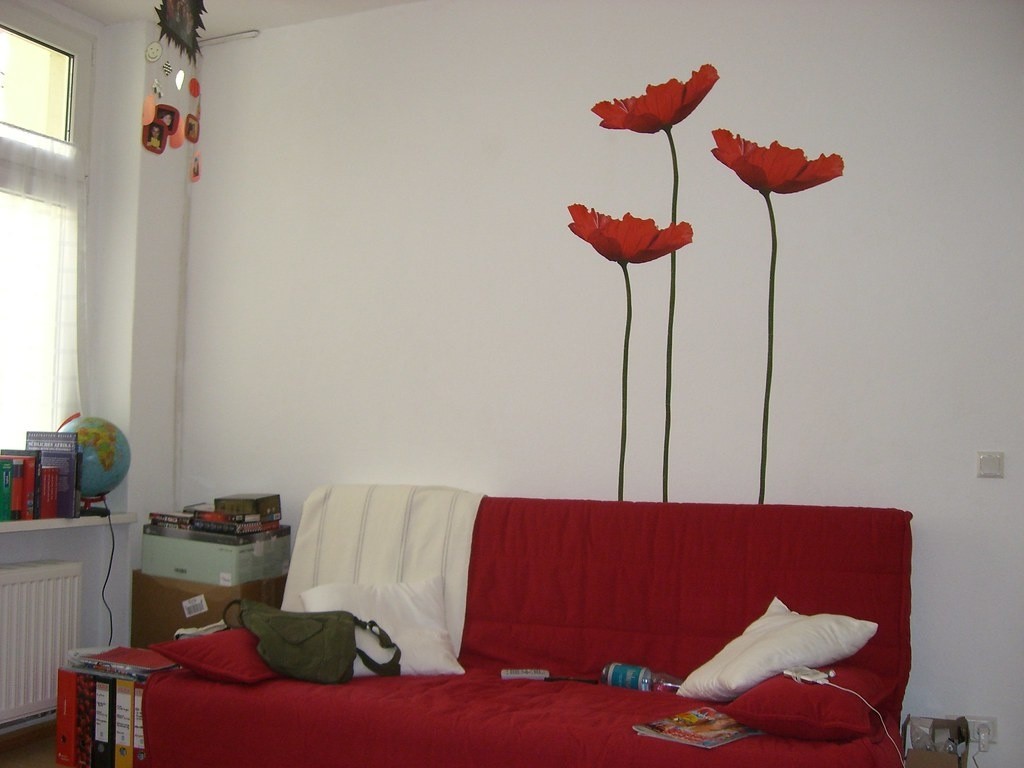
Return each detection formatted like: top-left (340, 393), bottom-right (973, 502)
top-left (0, 560), bottom-right (82, 737)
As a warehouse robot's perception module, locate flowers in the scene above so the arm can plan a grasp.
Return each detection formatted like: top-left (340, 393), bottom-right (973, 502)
top-left (568, 205), bottom-right (693, 502)
top-left (711, 127), bottom-right (845, 505)
top-left (591, 65), bottom-right (719, 502)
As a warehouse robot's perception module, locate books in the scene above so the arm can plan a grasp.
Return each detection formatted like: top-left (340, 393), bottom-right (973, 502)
top-left (632, 705), bottom-right (763, 748)
top-left (0, 431), bottom-right (85, 522)
top-left (143, 494), bottom-right (282, 536)
top-left (55, 646), bottom-right (176, 768)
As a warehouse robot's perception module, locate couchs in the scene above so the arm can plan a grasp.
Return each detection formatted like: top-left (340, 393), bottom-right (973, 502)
top-left (142, 484), bottom-right (914, 768)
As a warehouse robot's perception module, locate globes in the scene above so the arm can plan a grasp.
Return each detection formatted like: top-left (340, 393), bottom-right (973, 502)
top-left (55, 411), bottom-right (131, 518)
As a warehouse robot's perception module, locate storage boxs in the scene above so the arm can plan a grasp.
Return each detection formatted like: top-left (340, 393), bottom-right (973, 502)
top-left (142, 524), bottom-right (291, 587)
top-left (131, 569), bottom-right (288, 651)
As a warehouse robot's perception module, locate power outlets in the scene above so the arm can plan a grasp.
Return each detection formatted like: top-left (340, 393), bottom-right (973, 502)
top-left (943, 715), bottom-right (997, 743)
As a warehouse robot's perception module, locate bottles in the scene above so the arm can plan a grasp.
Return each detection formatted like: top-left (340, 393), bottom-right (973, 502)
top-left (601, 663), bottom-right (684, 696)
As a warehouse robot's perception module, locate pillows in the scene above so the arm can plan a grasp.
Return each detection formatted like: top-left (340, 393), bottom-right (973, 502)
top-left (300, 573), bottom-right (466, 676)
top-left (146, 628), bottom-right (278, 684)
top-left (714, 644), bottom-right (900, 741)
top-left (676, 595), bottom-right (879, 702)
